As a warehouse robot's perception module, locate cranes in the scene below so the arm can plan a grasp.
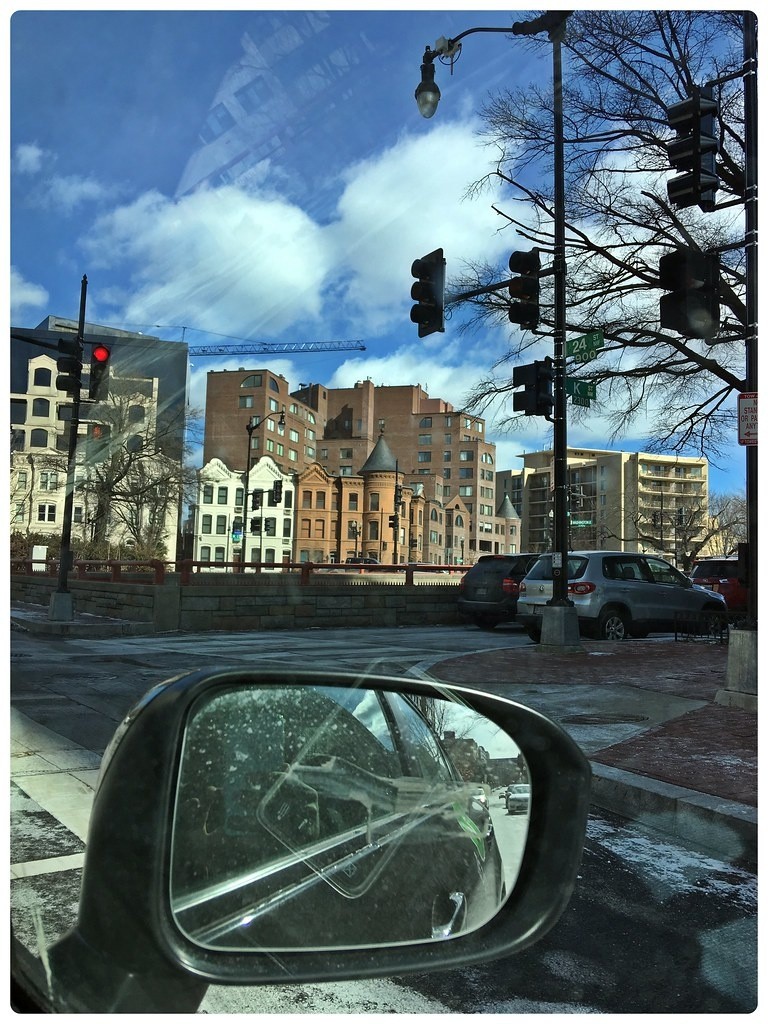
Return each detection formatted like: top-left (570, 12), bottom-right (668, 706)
top-left (86, 319), bottom-right (367, 357)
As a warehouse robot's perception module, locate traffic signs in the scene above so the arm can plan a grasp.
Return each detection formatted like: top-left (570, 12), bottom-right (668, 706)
top-left (735, 392), bottom-right (758, 447)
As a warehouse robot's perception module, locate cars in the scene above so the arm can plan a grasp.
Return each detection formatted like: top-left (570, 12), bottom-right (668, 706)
top-left (467, 787), bottom-right (490, 811)
top-left (498, 783), bottom-right (530, 815)
top-left (310, 557), bottom-right (445, 573)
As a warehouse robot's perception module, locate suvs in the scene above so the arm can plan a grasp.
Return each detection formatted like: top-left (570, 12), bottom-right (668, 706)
top-left (686, 557), bottom-right (746, 624)
top-left (456, 552), bottom-right (543, 630)
top-left (516, 549), bottom-right (729, 643)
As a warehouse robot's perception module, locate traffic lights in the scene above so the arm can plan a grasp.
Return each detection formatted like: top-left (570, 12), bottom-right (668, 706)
top-left (273, 480), bottom-right (283, 503)
top-left (54, 337), bottom-right (85, 398)
top-left (408, 248), bottom-right (446, 338)
top-left (508, 249), bottom-right (541, 331)
top-left (87, 342), bottom-right (114, 401)
top-left (657, 246), bottom-right (722, 339)
top-left (664, 84), bottom-right (721, 213)
top-left (250, 519), bottom-right (260, 533)
top-left (251, 491), bottom-right (260, 511)
top-left (511, 356), bottom-right (554, 417)
top-left (652, 512), bottom-right (660, 529)
top-left (264, 518), bottom-right (270, 532)
top-left (84, 424), bottom-right (111, 464)
top-left (388, 514), bottom-right (398, 528)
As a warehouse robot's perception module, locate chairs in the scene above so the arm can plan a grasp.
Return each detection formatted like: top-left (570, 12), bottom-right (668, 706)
top-left (621, 566), bottom-right (634, 580)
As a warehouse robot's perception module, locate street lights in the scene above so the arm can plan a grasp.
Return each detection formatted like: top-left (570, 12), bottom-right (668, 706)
top-left (351, 520), bottom-right (362, 557)
top-left (458, 534), bottom-right (466, 574)
top-left (240, 409), bottom-right (286, 573)
top-left (413, 9), bottom-right (582, 645)
